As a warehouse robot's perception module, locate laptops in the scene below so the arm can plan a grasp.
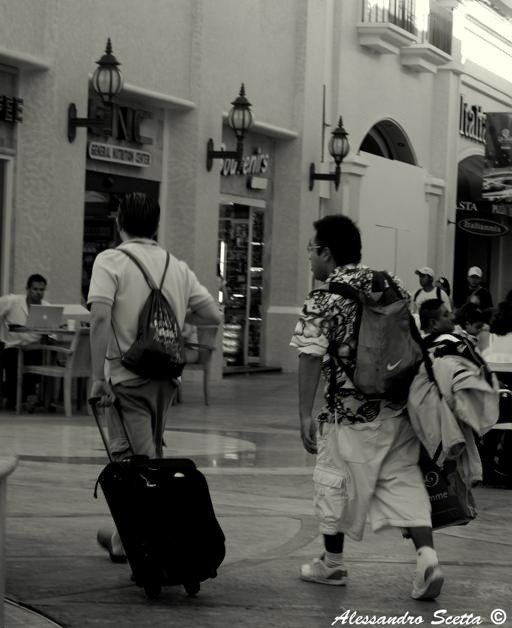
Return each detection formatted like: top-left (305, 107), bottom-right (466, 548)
top-left (15, 304), bottom-right (64, 331)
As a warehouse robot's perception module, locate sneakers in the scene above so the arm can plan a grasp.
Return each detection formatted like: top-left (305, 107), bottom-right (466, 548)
top-left (299, 552), bottom-right (348, 586)
top-left (98, 527), bottom-right (126, 564)
top-left (411, 558), bottom-right (444, 599)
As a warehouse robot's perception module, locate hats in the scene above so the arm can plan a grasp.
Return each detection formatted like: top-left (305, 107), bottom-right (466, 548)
top-left (468, 266), bottom-right (483, 278)
top-left (414, 267), bottom-right (434, 282)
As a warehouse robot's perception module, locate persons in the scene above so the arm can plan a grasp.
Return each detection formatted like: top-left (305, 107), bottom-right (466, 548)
top-left (287, 214), bottom-right (446, 602)
top-left (87, 192), bottom-right (221, 563)
top-left (408, 265), bottom-right (512, 364)
top-left (0, 274), bottom-right (53, 414)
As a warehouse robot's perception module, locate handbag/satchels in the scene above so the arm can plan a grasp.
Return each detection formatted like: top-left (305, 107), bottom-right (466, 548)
top-left (399, 443), bottom-right (478, 538)
top-left (424, 331), bottom-right (491, 395)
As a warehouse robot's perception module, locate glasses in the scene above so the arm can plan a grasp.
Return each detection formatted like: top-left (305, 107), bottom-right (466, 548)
top-left (307, 239), bottom-right (321, 251)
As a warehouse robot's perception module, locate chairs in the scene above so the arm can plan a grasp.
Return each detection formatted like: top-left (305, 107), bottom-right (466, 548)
top-left (182, 325), bottom-right (219, 405)
top-left (16, 328), bottom-right (91, 417)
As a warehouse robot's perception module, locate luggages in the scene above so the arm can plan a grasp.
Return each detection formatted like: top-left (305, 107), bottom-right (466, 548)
top-left (88, 394), bottom-right (225, 602)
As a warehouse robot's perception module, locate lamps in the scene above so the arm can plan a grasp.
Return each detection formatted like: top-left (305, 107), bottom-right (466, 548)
top-left (310, 116), bottom-right (350, 192)
top-left (68, 37), bottom-right (125, 142)
top-left (206, 82), bottom-right (253, 171)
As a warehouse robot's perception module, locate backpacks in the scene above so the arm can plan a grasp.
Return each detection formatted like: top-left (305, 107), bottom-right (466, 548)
top-left (308, 270), bottom-right (424, 393)
top-left (111, 248), bottom-right (187, 381)
